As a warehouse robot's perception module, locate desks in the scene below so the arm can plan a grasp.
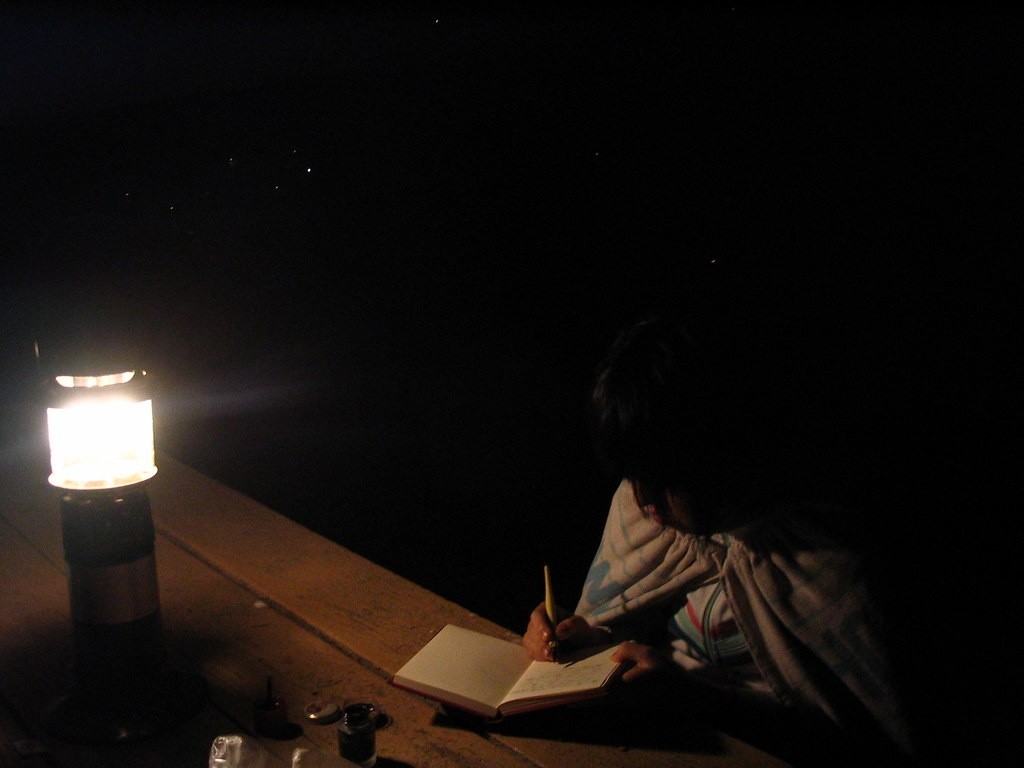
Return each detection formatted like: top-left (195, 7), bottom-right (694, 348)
top-left (2, 395), bottom-right (786, 768)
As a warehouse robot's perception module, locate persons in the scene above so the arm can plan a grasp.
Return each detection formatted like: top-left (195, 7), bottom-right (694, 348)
top-left (520, 305), bottom-right (936, 766)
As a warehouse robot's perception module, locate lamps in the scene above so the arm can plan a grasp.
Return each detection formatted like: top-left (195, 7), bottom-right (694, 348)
top-left (40, 356), bottom-right (167, 654)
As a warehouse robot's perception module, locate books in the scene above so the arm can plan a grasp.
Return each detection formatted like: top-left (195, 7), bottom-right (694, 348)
top-left (392, 617), bottom-right (648, 715)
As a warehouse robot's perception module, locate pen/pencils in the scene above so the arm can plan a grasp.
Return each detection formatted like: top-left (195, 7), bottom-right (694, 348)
top-left (543, 564), bottom-right (565, 664)
